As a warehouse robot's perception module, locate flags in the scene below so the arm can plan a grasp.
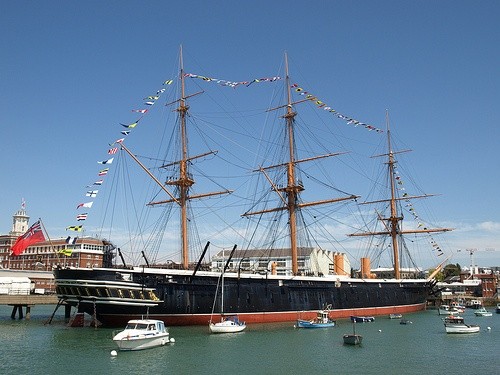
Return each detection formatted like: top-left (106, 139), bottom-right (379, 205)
top-left (107, 148), bottom-right (118, 155)
top-left (86, 190), bottom-right (98, 198)
top-left (77, 202), bottom-right (93, 209)
top-left (390, 151), bottom-right (444, 257)
top-left (291, 83), bottom-right (383, 132)
top-left (66, 224), bottom-right (85, 232)
top-left (65, 236), bottom-right (78, 245)
top-left (86, 179), bottom-right (104, 187)
top-left (99, 168), bottom-right (109, 176)
top-left (76, 213), bottom-right (88, 221)
top-left (59, 249), bottom-right (74, 256)
top-left (97, 157), bottom-right (114, 165)
top-left (184, 73), bottom-right (282, 89)
top-left (11, 220), bottom-right (45, 256)
top-left (109, 80), bottom-right (174, 146)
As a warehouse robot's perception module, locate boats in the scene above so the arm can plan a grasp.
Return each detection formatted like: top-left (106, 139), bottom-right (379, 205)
top-left (112, 306), bottom-right (169, 351)
top-left (343, 318), bottom-right (363, 345)
top-left (442, 313), bottom-right (480, 334)
top-left (297, 303), bottom-right (335, 327)
top-left (389, 313), bottom-right (402, 319)
top-left (439, 298), bottom-right (500, 317)
top-left (351, 315), bottom-right (375, 322)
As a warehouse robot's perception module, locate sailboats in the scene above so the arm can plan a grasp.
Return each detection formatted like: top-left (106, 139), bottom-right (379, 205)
top-left (51, 43), bottom-right (453, 328)
top-left (208, 249), bottom-right (247, 334)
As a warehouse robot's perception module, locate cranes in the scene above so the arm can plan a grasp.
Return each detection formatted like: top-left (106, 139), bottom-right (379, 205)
top-left (457, 247), bottom-right (496, 266)
